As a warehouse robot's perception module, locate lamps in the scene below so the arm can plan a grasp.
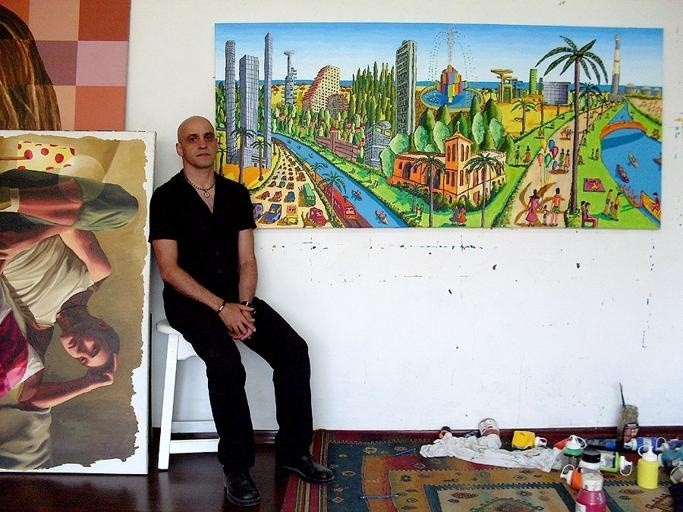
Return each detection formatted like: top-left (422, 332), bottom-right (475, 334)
top-left (0, 140), bottom-right (74, 175)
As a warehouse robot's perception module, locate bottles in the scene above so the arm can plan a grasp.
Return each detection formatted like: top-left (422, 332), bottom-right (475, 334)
top-left (560, 435), bottom-right (633, 491)
top-left (624, 437), bottom-right (683, 453)
top-left (575, 472), bottom-right (607, 512)
top-left (636, 444), bottom-right (660, 490)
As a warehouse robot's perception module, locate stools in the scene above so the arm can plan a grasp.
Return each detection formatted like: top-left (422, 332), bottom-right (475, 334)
top-left (156, 317), bottom-right (260, 470)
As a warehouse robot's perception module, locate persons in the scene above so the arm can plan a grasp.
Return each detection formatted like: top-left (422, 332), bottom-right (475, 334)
top-left (0, 163), bottom-right (142, 412)
top-left (147, 114), bottom-right (337, 509)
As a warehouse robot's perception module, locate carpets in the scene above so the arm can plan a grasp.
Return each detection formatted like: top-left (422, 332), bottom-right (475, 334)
top-left (282, 429), bottom-right (683, 512)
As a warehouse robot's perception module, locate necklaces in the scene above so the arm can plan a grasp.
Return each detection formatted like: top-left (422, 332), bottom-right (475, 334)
top-left (185, 176), bottom-right (215, 198)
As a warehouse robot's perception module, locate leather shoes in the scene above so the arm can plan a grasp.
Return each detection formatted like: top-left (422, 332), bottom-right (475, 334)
top-left (273, 454), bottom-right (337, 486)
top-left (222, 467), bottom-right (262, 509)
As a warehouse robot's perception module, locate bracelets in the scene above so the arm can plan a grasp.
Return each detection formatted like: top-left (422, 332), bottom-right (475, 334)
top-left (214, 300), bottom-right (230, 315)
top-left (240, 300), bottom-right (257, 316)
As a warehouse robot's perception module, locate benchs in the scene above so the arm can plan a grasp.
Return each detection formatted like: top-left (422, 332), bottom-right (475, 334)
top-left (580, 211), bottom-right (598, 228)
